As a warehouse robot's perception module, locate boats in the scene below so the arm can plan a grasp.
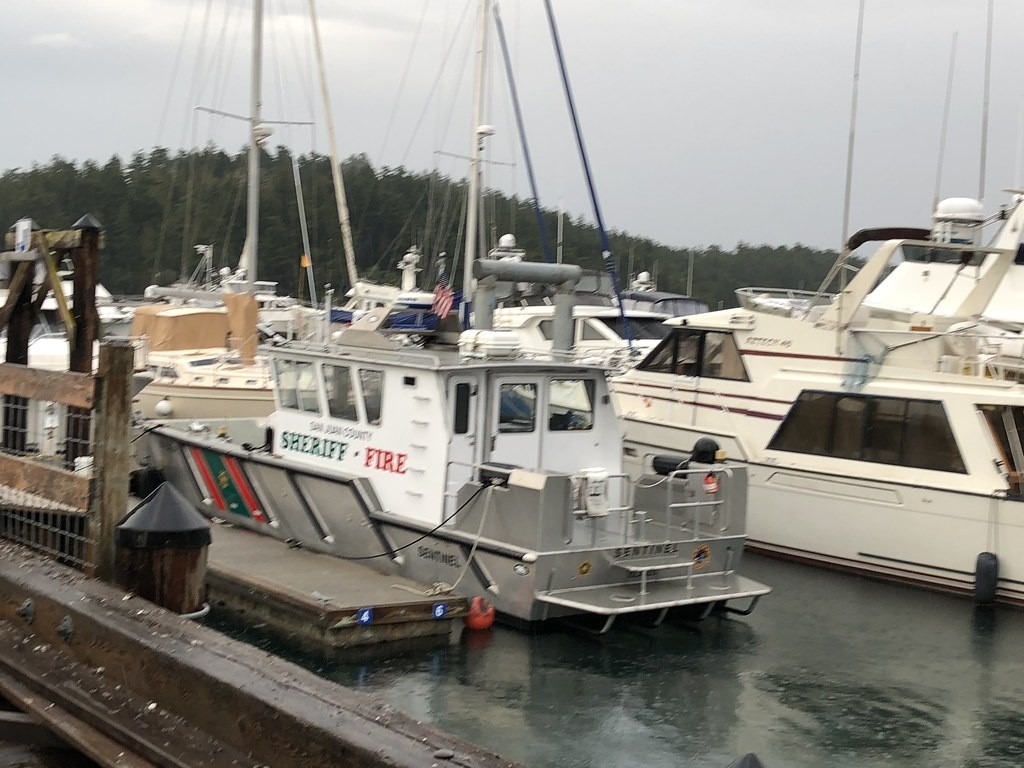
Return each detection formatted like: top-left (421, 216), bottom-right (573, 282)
top-left (2, 3), bottom-right (1024, 640)
